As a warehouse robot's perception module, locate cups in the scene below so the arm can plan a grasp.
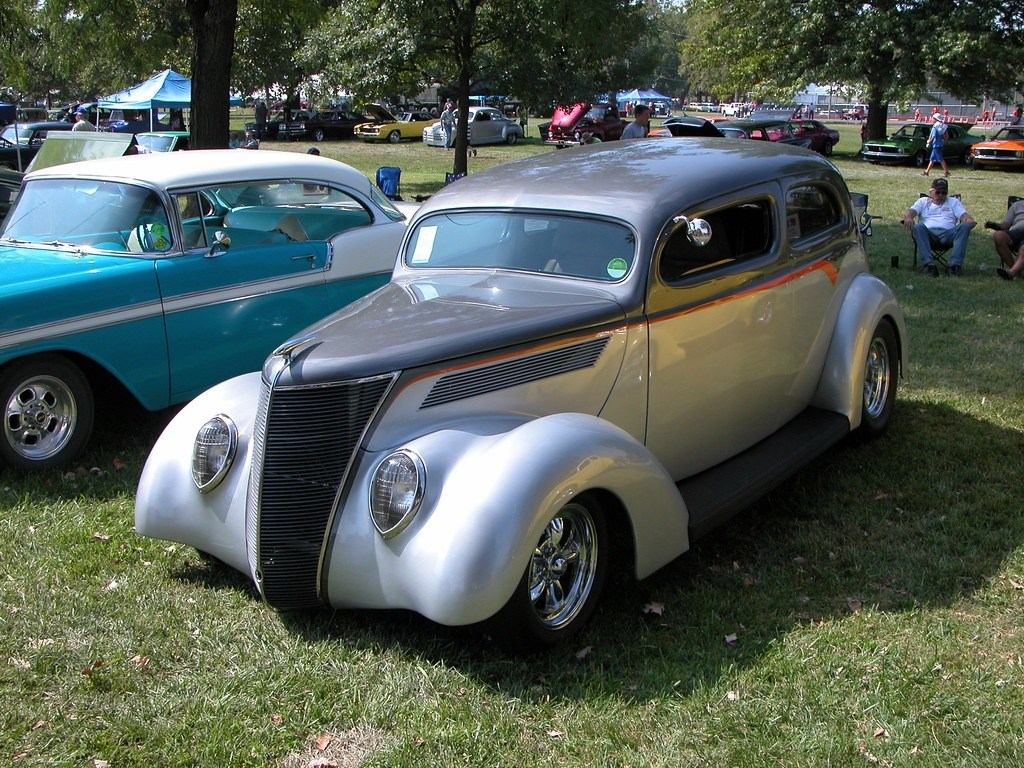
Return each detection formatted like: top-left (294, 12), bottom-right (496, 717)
top-left (891, 256), bottom-right (898, 269)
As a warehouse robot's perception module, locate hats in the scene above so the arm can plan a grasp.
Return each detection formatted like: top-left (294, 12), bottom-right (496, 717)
top-left (580, 132), bottom-right (593, 143)
top-left (933, 112), bottom-right (941, 121)
top-left (931, 178), bottom-right (948, 189)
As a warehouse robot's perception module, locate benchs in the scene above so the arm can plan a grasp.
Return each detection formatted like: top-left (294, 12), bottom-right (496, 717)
top-left (225, 205), bottom-right (372, 239)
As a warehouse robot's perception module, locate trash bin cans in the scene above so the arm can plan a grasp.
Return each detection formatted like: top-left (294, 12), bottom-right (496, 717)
top-left (377, 166), bottom-right (401, 196)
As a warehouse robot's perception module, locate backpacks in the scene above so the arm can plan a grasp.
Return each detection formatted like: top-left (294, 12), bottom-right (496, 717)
top-left (937, 123), bottom-right (949, 143)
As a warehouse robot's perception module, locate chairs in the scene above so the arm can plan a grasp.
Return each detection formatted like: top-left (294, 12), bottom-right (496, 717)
top-left (195, 226), bottom-right (288, 247)
top-left (376, 166), bottom-right (402, 200)
top-left (900, 190), bottom-right (977, 271)
top-left (444, 172), bottom-right (468, 185)
top-left (125, 224), bottom-right (202, 253)
top-left (849, 192), bottom-right (883, 248)
top-left (1000, 195), bottom-right (1024, 270)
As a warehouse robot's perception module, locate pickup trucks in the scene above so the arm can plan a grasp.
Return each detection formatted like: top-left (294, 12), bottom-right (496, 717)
top-left (843, 104), bottom-right (869, 118)
top-left (720, 103), bottom-right (743, 117)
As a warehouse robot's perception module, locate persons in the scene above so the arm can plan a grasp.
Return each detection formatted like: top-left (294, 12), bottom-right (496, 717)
top-left (254, 102), bottom-right (271, 142)
top-left (625, 99), bottom-right (688, 119)
top-left (440, 98), bottom-right (457, 151)
top-left (858, 107), bottom-right (864, 123)
top-left (738, 100), bottom-right (757, 118)
top-left (229, 131), bottom-right (258, 149)
top-left (307, 148), bottom-right (320, 156)
top-left (905, 178), bottom-right (976, 277)
top-left (580, 132), bottom-right (602, 146)
top-left (71, 107), bottom-right (95, 132)
top-left (1011, 108), bottom-right (1024, 125)
top-left (804, 103), bottom-right (814, 119)
top-left (497, 98), bottom-right (506, 115)
top-left (931, 107), bottom-right (949, 121)
top-left (984, 200), bottom-right (1024, 279)
top-left (914, 108), bottom-right (920, 121)
top-left (124, 141), bottom-right (151, 155)
top-left (516, 103), bottom-right (527, 139)
top-left (921, 112), bottom-right (950, 178)
top-left (620, 105), bottom-right (650, 140)
top-left (984, 108), bottom-right (996, 121)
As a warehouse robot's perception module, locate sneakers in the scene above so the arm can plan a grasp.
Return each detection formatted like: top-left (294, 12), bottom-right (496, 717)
top-left (946, 269), bottom-right (959, 280)
top-left (926, 267), bottom-right (939, 279)
top-left (997, 268), bottom-right (1014, 281)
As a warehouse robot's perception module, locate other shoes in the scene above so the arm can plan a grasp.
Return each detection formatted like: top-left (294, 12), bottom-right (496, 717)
top-left (919, 171), bottom-right (928, 177)
top-left (469, 149), bottom-right (477, 157)
top-left (941, 171), bottom-right (951, 177)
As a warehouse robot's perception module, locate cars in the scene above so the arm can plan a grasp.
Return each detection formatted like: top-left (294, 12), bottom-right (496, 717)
top-left (544, 102), bottom-right (631, 150)
top-left (423, 107), bottom-right (523, 147)
top-left (277, 110), bottom-right (371, 141)
top-left (970, 125), bottom-right (1024, 171)
top-left (0, 149), bottom-right (437, 481)
top-left (819, 110), bottom-right (839, 118)
top-left (0, 122), bottom-right (78, 172)
top-left (13, 107), bottom-right (75, 122)
top-left (653, 103), bottom-right (670, 114)
top-left (682, 102), bottom-right (725, 112)
top-left (245, 109), bottom-right (314, 141)
top-left (354, 102), bottom-right (441, 143)
top-left (648, 104), bottom-right (840, 156)
top-left (134, 131), bottom-right (190, 154)
top-left (134, 136), bottom-right (910, 658)
top-left (860, 121), bottom-right (986, 167)
top-left (0, 128), bottom-right (139, 222)
top-left (106, 111), bottom-right (171, 133)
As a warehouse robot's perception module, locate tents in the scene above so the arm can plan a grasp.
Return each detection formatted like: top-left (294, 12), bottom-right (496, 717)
top-left (97, 69), bottom-right (246, 133)
top-left (595, 89), bottom-right (671, 118)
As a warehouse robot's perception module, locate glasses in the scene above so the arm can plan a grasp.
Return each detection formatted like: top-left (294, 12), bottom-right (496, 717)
top-left (934, 191), bottom-right (947, 196)
